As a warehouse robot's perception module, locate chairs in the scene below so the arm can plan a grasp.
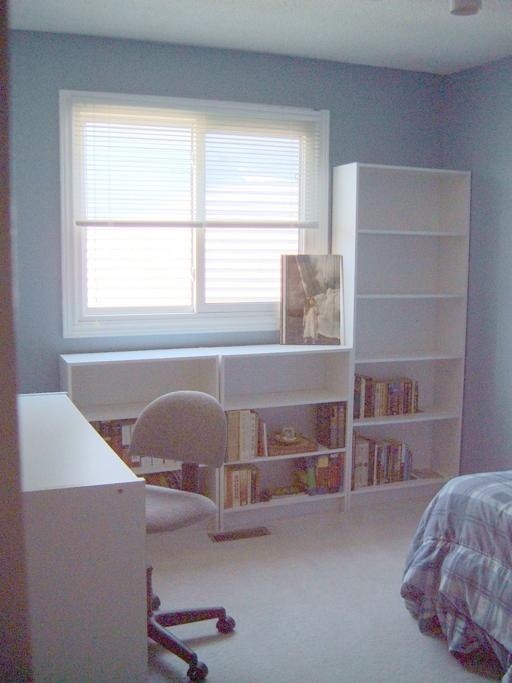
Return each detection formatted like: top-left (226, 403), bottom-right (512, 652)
top-left (125, 391), bottom-right (236, 682)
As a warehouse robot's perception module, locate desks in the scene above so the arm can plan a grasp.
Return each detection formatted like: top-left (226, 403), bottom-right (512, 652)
top-left (17, 389), bottom-right (148, 683)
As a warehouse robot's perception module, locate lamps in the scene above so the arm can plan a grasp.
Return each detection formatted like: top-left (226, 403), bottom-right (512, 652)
top-left (449, 0), bottom-right (482, 16)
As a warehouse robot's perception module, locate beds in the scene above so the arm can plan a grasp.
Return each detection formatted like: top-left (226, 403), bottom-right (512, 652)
top-left (400, 470), bottom-right (512, 683)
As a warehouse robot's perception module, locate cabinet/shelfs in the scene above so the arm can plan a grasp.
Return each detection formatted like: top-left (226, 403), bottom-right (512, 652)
top-left (59, 344), bottom-right (351, 532)
top-left (332, 160), bottom-right (472, 513)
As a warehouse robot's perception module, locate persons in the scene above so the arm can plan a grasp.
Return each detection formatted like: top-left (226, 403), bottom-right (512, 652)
top-left (301, 296), bottom-right (321, 345)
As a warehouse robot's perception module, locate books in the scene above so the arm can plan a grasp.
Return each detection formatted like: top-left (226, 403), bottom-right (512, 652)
top-left (92, 418), bottom-right (203, 495)
top-left (217, 399), bottom-right (345, 510)
top-left (350, 370), bottom-right (419, 491)
